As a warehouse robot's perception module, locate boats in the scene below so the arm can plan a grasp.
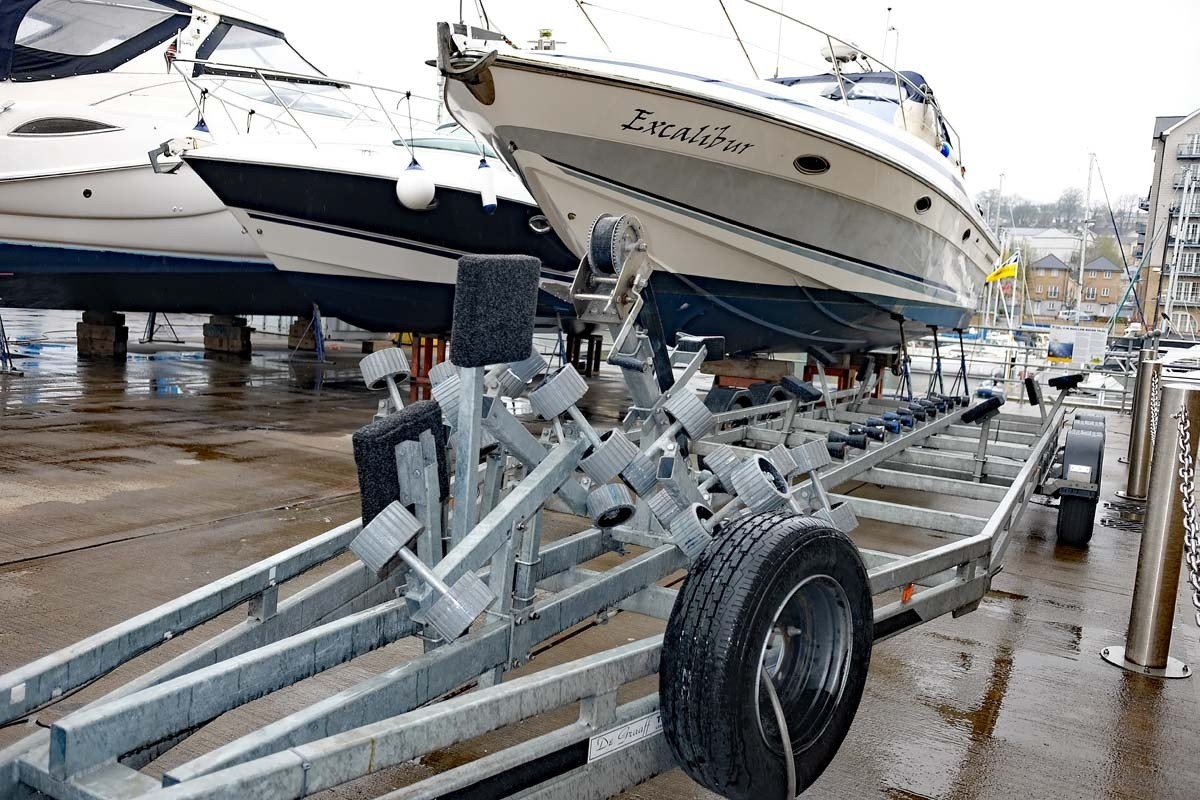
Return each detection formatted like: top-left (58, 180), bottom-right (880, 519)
top-left (441, 0), bottom-right (1003, 365)
top-left (0, 0), bottom-right (600, 336)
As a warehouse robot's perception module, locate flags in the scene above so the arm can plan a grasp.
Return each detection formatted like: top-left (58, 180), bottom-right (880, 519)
top-left (986, 252), bottom-right (1018, 283)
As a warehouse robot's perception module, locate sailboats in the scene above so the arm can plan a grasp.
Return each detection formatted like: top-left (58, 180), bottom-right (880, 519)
top-left (900, 152), bottom-right (1052, 378)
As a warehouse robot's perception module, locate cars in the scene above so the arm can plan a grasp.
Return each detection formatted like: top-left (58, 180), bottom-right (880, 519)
top-left (1055, 310), bottom-right (1090, 321)
